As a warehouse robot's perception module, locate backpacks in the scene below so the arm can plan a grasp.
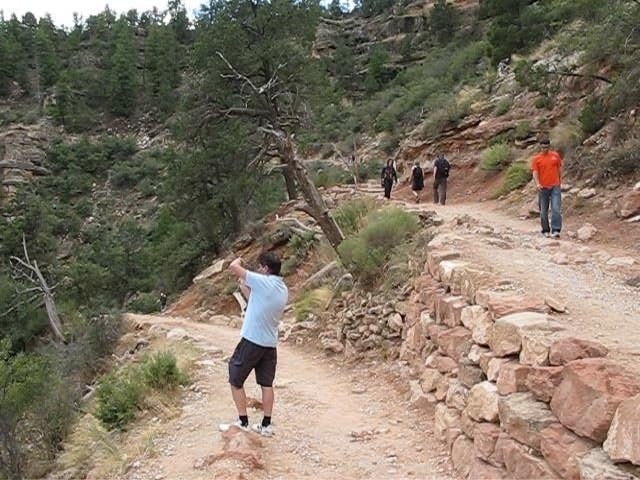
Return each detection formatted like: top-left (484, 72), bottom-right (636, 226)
top-left (438, 159), bottom-right (450, 178)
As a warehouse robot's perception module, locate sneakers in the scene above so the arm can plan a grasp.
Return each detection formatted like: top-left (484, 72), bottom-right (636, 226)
top-left (415, 196), bottom-right (422, 204)
top-left (551, 231), bottom-right (560, 238)
top-left (543, 232), bottom-right (550, 238)
top-left (433, 201), bottom-right (445, 206)
top-left (251, 423), bottom-right (273, 437)
top-left (220, 419), bottom-right (250, 433)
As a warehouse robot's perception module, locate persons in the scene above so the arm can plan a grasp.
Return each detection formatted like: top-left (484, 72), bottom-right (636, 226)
top-left (531, 139), bottom-right (563, 238)
top-left (408, 160), bottom-right (425, 203)
top-left (381, 158), bottom-right (398, 199)
top-left (432, 153), bottom-right (447, 205)
top-left (217, 251), bottom-right (289, 437)
